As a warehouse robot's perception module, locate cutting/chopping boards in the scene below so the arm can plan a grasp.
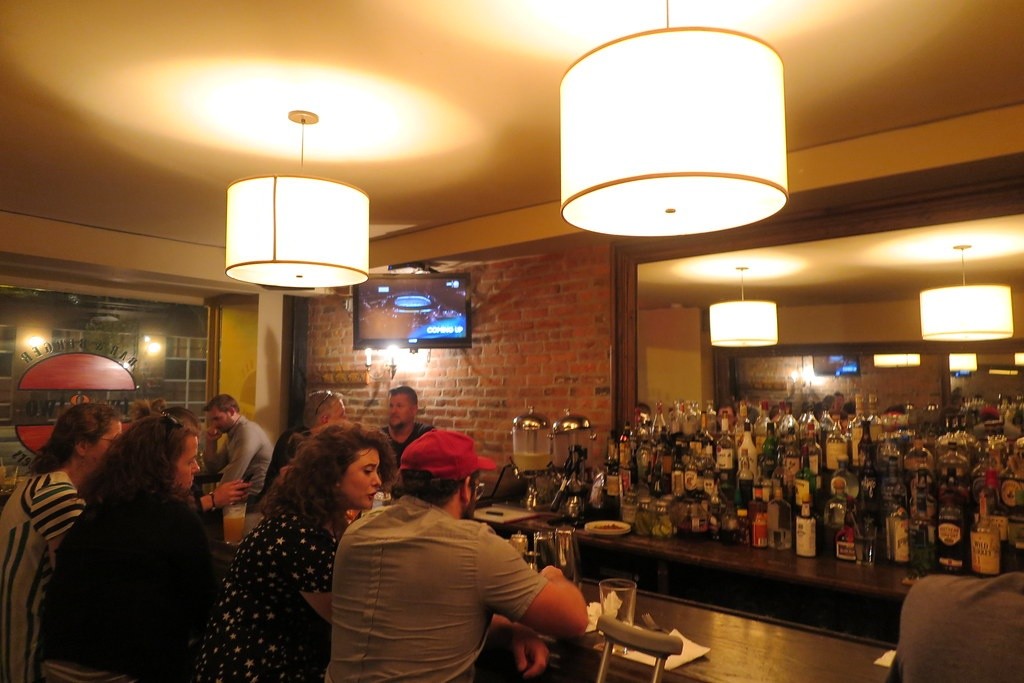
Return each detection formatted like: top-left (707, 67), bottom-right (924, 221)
top-left (472, 505), bottom-right (540, 524)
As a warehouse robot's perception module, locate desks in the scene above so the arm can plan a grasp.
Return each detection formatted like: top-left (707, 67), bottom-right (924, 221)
top-left (0, 470), bottom-right (944, 683)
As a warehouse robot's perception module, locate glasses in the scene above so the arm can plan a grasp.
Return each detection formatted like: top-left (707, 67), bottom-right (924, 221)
top-left (163, 411), bottom-right (180, 439)
top-left (315, 388), bottom-right (332, 416)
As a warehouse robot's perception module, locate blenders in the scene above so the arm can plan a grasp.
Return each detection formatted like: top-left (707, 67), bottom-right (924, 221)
top-left (509, 405), bottom-right (597, 529)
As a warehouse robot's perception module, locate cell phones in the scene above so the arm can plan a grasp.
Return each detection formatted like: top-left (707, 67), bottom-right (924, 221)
top-left (238, 473), bottom-right (254, 491)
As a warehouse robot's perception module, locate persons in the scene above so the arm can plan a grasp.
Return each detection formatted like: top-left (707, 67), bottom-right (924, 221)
top-left (253, 392), bottom-right (347, 511)
top-left (325, 428), bottom-right (588, 683)
top-left (193, 416), bottom-right (397, 683)
top-left (381, 386), bottom-right (432, 498)
top-left (883, 569), bottom-right (1024, 683)
top-left (638, 392), bottom-right (907, 431)
top-left (34, 416), bottom-right (211, 683)
top-left (130, 398), bottom-right (248, 513)
top-left (0, 403), bottom-right (122, 683)
top-left (952, 395), bottom-right (1021, 433)
top-left (202, 395), bottom-right (273, 506)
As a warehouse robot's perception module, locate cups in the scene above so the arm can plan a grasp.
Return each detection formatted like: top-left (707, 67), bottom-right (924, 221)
top-left (600, 578), bottom-right (637, 626)
top-left (222, 501), bottom-right (247, 546)
top-left (508, 529), bottom-right (578, 583)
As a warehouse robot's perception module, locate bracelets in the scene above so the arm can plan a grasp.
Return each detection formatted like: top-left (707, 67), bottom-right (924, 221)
top-left (209, 492), bottom-right (216, 511)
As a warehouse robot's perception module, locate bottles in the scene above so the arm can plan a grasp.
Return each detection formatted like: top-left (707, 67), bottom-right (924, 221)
top-left (605, 392), bottom-right (1024, 577)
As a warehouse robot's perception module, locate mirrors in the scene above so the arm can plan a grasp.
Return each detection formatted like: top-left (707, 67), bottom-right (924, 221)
top-left (610, 152), bottom-right (1024, 444)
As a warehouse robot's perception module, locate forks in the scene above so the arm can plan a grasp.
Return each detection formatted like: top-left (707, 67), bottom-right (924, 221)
top-left (642, 613), bottom-right (669, 634)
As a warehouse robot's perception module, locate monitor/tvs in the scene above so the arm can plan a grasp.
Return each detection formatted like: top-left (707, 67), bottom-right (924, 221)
top-left (812, 355), bottom-right (861, 376)
top-left (353, 272), bottom-right (472, 350)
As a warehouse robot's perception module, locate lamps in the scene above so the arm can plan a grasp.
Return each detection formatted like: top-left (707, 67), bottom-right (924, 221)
top-left (709, 267), bottom-right (778, 347)
top-left (920, 244), bottom-right (1014, 341)
top-left (559, 0), bottom-right (788, 236)
top-left (225, 110), bottom-right (369, 288)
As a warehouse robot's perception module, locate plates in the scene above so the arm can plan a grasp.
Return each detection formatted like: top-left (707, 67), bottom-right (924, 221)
top-left (584, 520), bottom-right (631, 537)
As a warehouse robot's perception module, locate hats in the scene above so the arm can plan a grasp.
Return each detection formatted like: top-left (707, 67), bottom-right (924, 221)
top-left (400, 427), bottom-right (495, 482)
